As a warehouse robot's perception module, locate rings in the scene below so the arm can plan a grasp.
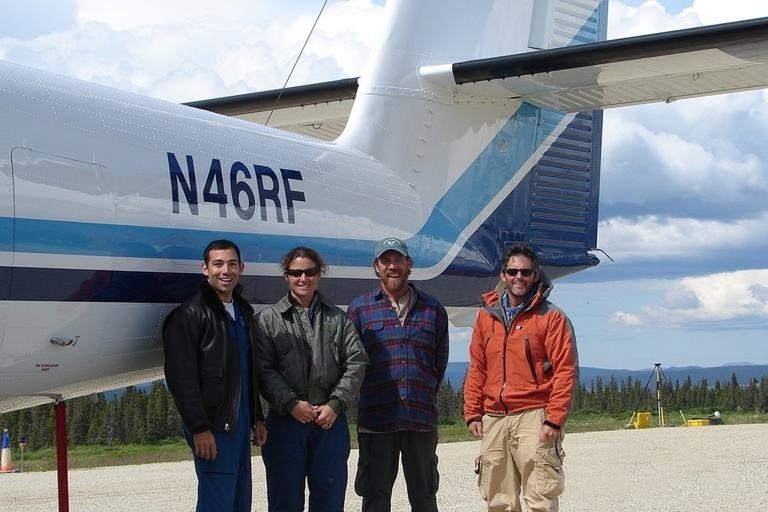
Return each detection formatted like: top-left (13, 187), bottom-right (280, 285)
top-left (326, 421), bottom-right (329, 426)
top-left (200, 448), bottom-right (205, 453)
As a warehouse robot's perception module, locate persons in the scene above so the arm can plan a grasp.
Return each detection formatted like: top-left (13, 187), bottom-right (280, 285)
top-left (250, 245), bottom-right (369, 511)
top-left (160, 237), bottom-right (271, 511)
top-left (461, 242), bottom-right (582, 511)
top-left (344, 237), bottom-right (450, 510)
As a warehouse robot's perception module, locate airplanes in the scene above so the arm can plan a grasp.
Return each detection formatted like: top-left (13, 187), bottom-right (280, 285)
top-left (0, 0), bottom-right (768, 420)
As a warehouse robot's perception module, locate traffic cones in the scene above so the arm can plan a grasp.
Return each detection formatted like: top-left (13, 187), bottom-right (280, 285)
top-left (0, 427), bottom-right (20, 474)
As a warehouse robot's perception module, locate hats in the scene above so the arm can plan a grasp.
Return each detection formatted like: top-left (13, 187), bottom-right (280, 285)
top-left (375, 237), bottom-right (409, 260)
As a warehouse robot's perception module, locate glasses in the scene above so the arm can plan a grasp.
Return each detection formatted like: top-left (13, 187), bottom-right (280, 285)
top-left (503, 268), bottom-right (537, 276)
top-left (286, 266), bottom-right (321, 279)
